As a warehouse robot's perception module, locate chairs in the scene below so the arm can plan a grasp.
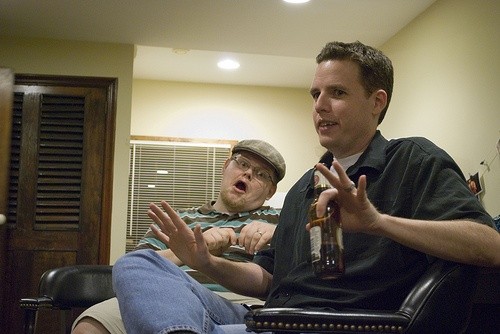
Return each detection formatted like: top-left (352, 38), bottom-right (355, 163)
top-left (19, 216), bottom-right (500, 334)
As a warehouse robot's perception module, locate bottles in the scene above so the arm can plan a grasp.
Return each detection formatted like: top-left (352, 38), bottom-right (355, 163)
top-left (309, 163), bottom-right (345, 280)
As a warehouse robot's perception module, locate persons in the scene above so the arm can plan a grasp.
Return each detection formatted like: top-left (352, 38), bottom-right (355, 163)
top-left (111, 37), bottom-right (500, 334)
top-left (68, 139), bottom-right (286, 334)
top-left (468, 179), bottom-right (477, 195)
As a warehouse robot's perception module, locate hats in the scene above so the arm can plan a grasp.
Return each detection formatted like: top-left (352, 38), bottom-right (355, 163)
top-left (231, 139), bottom-right (286, 182)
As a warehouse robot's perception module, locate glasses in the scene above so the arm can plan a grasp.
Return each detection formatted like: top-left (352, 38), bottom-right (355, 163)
top-left (231, 156), bottom-right (276, 185)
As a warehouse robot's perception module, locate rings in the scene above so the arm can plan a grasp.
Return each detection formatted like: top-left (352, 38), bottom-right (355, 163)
top-left (341, 181), bottom-right (355, 193)
top-left (257, 230), bottom-right (263, 237)
